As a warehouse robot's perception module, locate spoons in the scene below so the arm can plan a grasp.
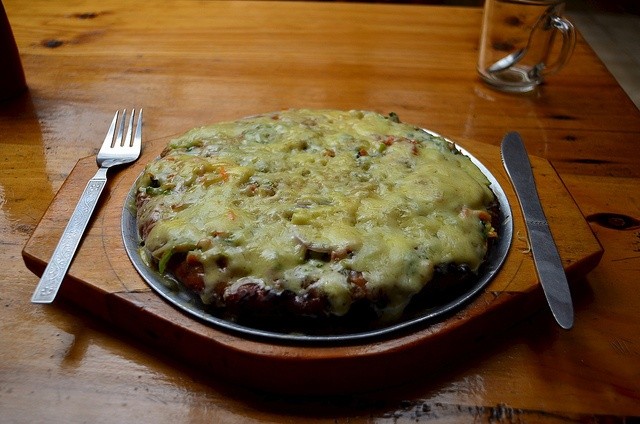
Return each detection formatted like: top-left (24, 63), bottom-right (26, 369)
top-left (488, 0), bottom-right (564, 73)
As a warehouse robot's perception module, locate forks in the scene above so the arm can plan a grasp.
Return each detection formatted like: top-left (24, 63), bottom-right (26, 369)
top-left (29, 108), bottom-right (143, 305)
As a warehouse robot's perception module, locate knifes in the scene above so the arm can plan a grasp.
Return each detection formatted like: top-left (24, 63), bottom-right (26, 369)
top-left (500, 131), bottom-right (575, 331)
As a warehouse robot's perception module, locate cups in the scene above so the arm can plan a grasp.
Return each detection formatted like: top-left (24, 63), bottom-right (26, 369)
top-left (475, 0), bottom-right (576, 96)
